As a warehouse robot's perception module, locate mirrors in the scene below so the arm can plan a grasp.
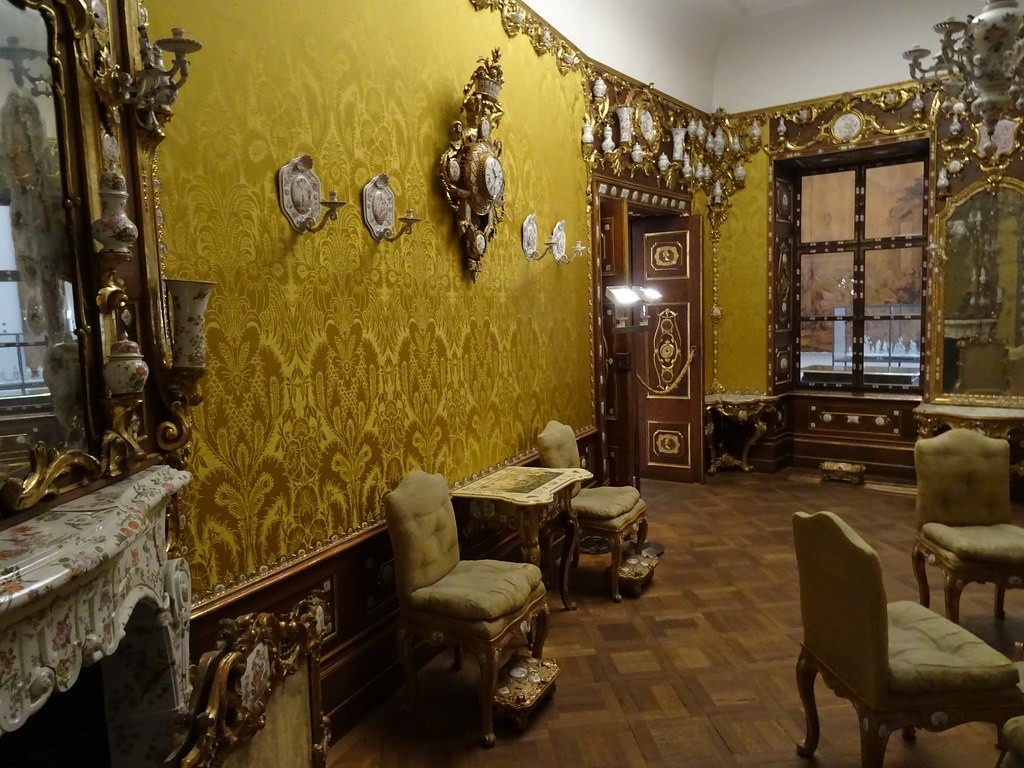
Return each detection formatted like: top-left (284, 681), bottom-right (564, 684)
top-left (1, 0), bottom-right (97, 489)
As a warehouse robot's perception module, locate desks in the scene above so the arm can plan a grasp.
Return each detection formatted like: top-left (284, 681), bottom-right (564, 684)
top-left (0, 465), bottom-right (194, 735)
top-left (705, 394), bottom-right (775, 475)
top-left (451, 465), bottom-right (595, 610)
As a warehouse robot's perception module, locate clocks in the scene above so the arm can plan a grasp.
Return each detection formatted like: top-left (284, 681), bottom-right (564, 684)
top-left (440, 47), bottom-right (505, 285)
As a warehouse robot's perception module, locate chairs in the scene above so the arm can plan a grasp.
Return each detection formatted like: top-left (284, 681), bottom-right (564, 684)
top-left (537, 421), bottom-right (648, 604)
top-left (792, 511), bottom-right (1024, 768)
top-left (156, 598), bottom-right (330, 768)
top-left (384, 471), bottom-right (552, 750)
top-left (911, 428), bottom-right (1024, 627)
